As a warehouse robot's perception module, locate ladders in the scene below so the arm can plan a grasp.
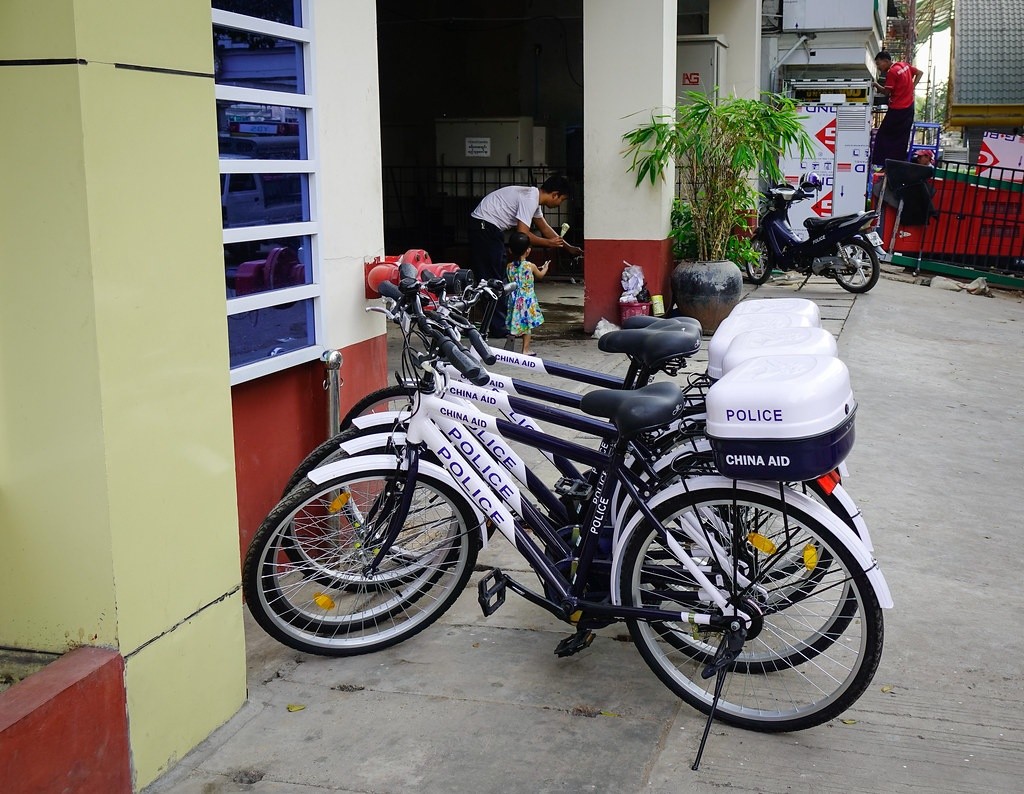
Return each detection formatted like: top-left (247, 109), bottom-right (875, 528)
top-left (908, 122), bottom-right (941, 169)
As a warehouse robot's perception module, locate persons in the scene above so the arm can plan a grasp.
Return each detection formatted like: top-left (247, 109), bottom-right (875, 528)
top-left (915, 149), bottom-right (935, 168)
top-left (505, 232), bottom-right (548, 355)
top-left (470, 175), bottom-right (583, 338)
top-left (871, 51), bottom-right (923, 166)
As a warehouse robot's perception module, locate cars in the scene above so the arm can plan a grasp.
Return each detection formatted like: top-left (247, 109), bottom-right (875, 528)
top-left (222, 119), bottom-right (304, 230)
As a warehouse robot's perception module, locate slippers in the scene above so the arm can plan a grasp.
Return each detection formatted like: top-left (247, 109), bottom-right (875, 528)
top-left (529, 350), bottom-right (536, 356)
top-left (500, 330), bottom-right (520, 339)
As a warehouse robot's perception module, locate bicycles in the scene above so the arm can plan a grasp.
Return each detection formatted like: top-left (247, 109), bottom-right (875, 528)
top-left (240, 253), bottom-right (896, 771)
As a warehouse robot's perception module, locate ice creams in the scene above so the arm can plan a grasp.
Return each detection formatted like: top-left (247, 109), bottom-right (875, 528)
top-left (561, 223), bottom-right (570, 237)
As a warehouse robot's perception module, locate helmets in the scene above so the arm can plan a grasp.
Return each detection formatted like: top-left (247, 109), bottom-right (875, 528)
top-left (799, 172), bottom-right (823, 192)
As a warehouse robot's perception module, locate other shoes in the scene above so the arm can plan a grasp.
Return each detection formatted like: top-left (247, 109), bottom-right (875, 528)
top-left (877, 167), bottom-right (886, 173)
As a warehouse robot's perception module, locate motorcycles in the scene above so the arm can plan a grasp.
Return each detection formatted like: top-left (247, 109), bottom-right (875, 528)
top-left (744, 173), bottom-right (888, 295)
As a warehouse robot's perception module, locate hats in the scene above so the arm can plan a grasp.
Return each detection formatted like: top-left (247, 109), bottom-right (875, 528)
top-left (916, 150), bottom-right (935, 161)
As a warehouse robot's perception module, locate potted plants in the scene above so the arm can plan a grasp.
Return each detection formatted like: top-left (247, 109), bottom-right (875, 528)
top-left (620, 79), bottom-right (823, 335)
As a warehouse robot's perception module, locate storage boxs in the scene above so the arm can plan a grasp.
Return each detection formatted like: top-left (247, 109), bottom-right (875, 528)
top-left (706, 297), bottom-right (858, 484)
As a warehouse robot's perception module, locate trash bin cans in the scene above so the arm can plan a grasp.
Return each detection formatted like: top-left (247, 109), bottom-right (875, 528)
top-left (619, 302), bottom-right (651, 323)
